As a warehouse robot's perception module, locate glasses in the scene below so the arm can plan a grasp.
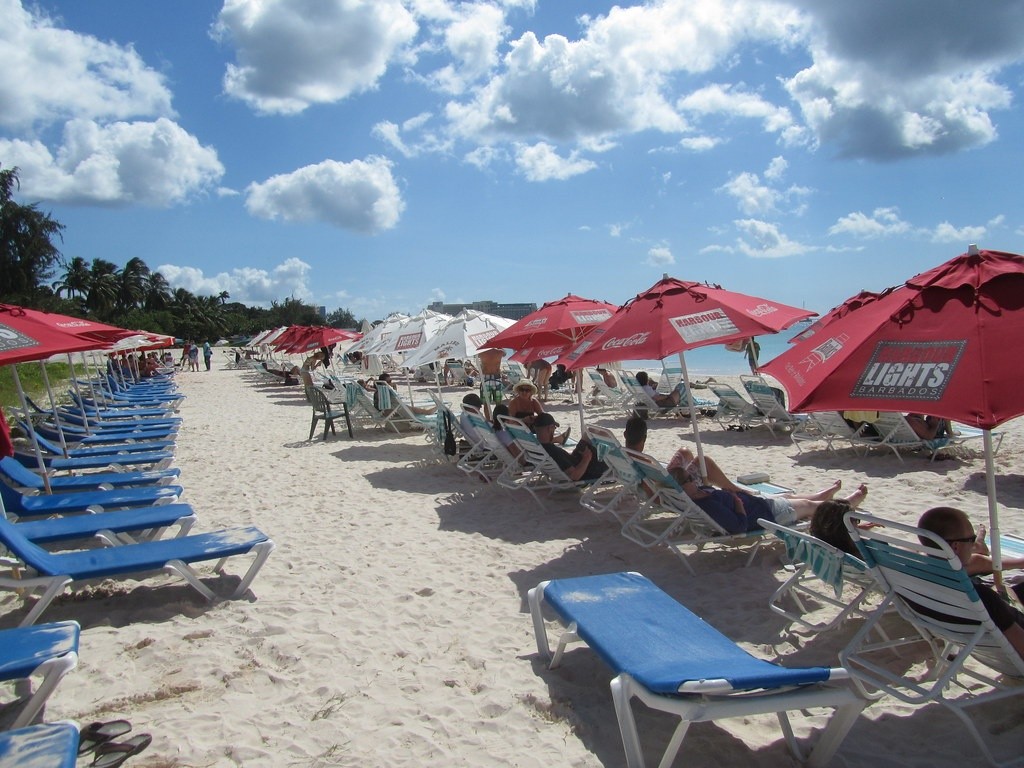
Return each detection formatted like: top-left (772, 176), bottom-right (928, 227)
top-left (682, 474), bottom-right (693, 485)
top-left (518, 386), bottom-right (531, 392)
top-left (950, 535), bottom-right (977, 543)
top-left (854, 518), bottom-right (860, 524)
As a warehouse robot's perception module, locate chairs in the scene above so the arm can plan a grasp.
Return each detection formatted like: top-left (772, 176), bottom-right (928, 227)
top-left (0, 347), bottom-right (1024, 768)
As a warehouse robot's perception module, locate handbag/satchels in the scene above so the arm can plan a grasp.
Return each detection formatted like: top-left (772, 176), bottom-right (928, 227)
top-left (444, 432), bottom-right (456, 455)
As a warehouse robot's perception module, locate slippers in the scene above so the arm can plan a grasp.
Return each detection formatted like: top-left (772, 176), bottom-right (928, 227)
top-left (89, 733), bottom-right (153, 767)
top-left (77, 719), bottom-right (133, 757)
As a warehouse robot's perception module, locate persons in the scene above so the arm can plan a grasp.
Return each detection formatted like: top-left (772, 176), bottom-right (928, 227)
top-left (107, 340), bottom-right (212, 376)
top-left (666, 466), bottom-right (869, 537)
top-left (809, 499), bottom-right (1024, 575)
top-left (837, 410), bottom-right (880, 437)
top-left (235, 335), bottom-right (761, 490)
top-left (918, 508), bottom-right (1024, 661)
top-left (902, 412), bottom-right (961, 440)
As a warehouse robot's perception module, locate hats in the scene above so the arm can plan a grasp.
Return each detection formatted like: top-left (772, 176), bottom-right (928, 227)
top-left (512, 378), bottom-right (537, 395)
top-left (463, 393), bottom-right (482, 405)
top-left (534, 412), bottom-right (561, 427)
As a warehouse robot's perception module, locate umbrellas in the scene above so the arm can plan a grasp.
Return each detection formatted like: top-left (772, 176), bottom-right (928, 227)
top-left (347, 307), bottom-right (520, 421)
top-left (479, 244), bottom-right (1024, 593)
top-left (214, 324), bottom-right (363, 375)
top-left (0, 302), bottom-right (176, 522)
